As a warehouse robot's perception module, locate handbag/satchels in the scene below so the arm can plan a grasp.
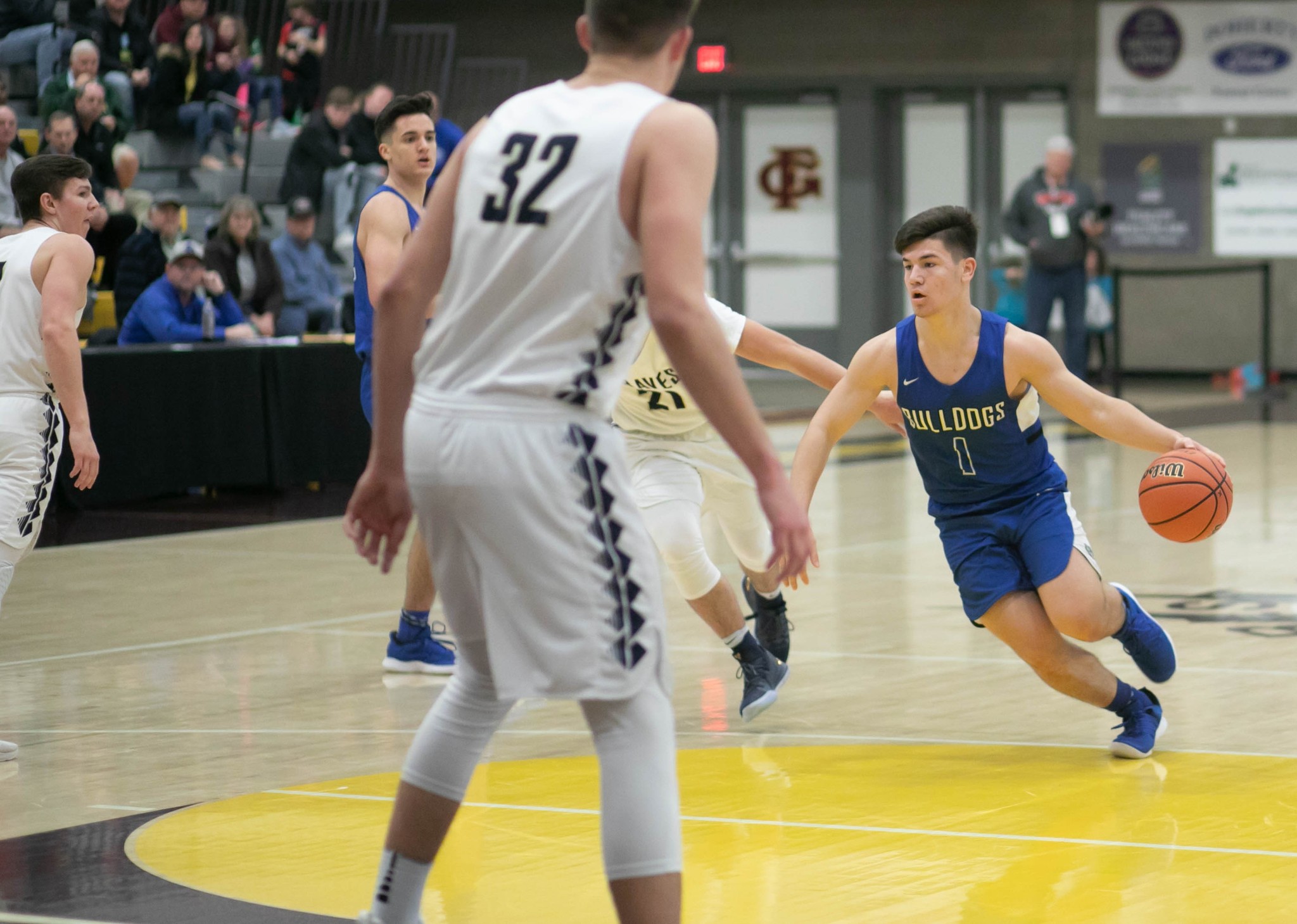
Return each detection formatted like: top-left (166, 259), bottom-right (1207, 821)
top-left (1050, 273), bottom-right (1114, 332)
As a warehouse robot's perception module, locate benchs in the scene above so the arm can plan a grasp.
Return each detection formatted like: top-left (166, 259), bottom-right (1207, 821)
top-left (122, 120), bottom-right (304, 243)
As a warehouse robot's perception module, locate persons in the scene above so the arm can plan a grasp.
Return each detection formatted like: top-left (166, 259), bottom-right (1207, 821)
top-left (352, 90), bottom-right (456, 674)
top-left (0, 0), bottom-right (464, 348)
top-left (987, 134), bottom-right (1117, 381)
top-left (344, 1), bottom-right (819, 924)
top-left (779, 204), bottom-right (1228, 760)
top-left (0, 153), bottom-right (98, 760)
top-left (606, 289), bottom-right (908, 722)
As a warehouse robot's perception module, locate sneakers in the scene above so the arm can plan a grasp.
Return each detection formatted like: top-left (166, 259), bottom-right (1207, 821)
top-left (732, 644), bottom-right (790, 722)
top-left (381, 621), bottom-right (458, 675)
top-left (1110, 581), bottom-right (1177, 684)
top-left (741, 576), bottom-right (794, 663)
top-left (1111, 686), bottom-right (1167, 759)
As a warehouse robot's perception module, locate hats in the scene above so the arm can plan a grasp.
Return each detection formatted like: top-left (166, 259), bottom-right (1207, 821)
top-left (168, 239), bottom-right (208, 265)
top-left (151, 190), bottom-right (186, 209)
top-left (286, 196), bottom-right (316, 221)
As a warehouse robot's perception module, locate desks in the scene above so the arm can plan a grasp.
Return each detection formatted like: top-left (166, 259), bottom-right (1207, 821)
top-left (47, 333), bottom-right (370, 545)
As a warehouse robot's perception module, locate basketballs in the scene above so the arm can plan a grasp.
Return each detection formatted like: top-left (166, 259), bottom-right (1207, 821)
top-left (1138, 449), bottom-right (1233, 544)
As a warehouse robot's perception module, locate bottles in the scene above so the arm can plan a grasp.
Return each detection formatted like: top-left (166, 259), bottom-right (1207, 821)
top-left (202, 299), bottom-right (214, 341)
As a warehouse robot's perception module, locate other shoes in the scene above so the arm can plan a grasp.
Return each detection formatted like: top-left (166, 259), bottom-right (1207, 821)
top-left (228, 156), bottom-right (243, 168)
top-left (268, 119), bottom-right (299, 140)
top-left (1, 741), bottom-right (18, 763)
top-left (197, 157), bottom-right (223, 173)
